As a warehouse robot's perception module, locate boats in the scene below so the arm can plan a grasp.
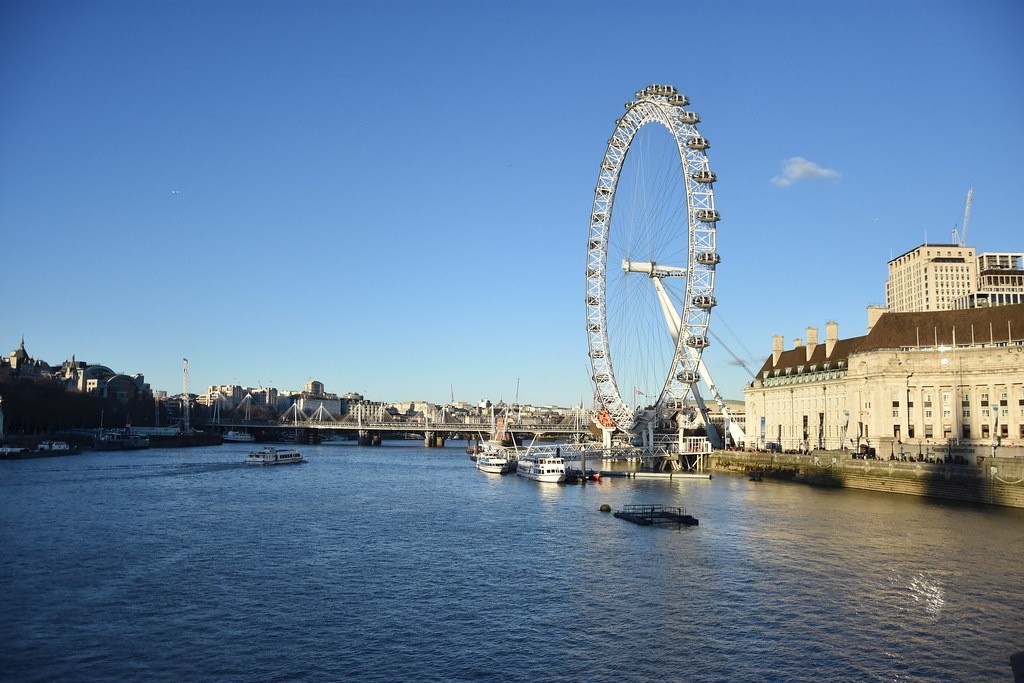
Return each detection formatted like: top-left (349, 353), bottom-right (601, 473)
top-left (92, 422), bottom-right (152, 450)
top-left (514, 448), bottom-right (567, 486)
top-left (247, 446), bottom-right (308, 468)
top-left (474, 440), bottom-right (512, 475)
top-left (220, 428), bottom-right (255, 444)
top-left (579, 467), bottom-right (602, 481)
top-left (2, 445), bottom-right (31, 458)
top-left (37, 439), bottom-right (74, 455)
